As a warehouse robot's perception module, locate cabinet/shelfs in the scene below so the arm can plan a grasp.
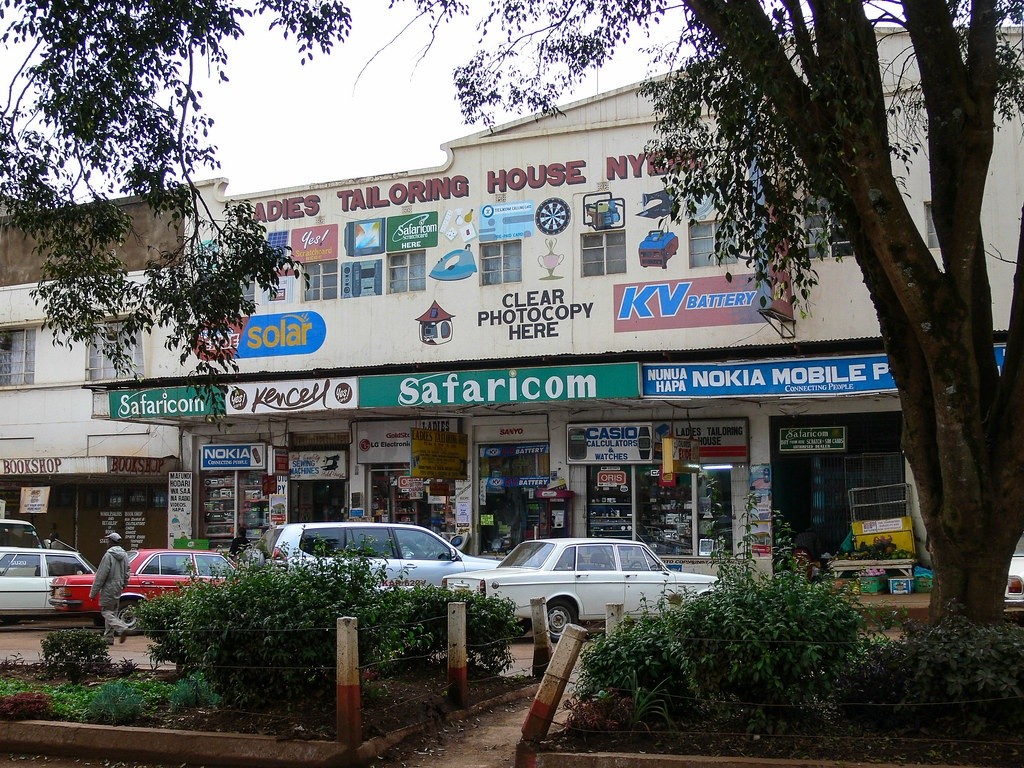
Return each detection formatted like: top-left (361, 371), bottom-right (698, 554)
top-left (642, 472), bottom-right (715, 555)
top-left (392, 486), bottom-right (420, 526)
top-left (587, 503), bottom-right (632, 541)
top-left (205, 473), bottom-right (269, 538)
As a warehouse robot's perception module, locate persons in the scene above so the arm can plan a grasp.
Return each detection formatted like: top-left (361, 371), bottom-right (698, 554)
top-left (90, 532), bottom-right (130, 646)
top-left (229, 527), bottom-right (249, 560)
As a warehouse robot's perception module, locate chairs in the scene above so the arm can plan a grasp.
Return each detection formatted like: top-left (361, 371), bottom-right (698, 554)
top-left (589, 552), bottom-right (609, 570)
top-left (562, 552), bottom-right (583, 567)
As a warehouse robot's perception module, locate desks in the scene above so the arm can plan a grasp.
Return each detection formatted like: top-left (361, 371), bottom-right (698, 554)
top-left (830, 558), bottom-right (918, 578)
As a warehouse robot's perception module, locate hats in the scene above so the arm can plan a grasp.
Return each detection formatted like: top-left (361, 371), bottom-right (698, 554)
top-left (238, 527), bottom-right (246, 535)
top-left (105, 532), bottom-right (121, 542)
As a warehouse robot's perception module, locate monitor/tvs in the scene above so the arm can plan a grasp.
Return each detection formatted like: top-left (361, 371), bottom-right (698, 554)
top-left (344, 218), bottom-right (385, 257)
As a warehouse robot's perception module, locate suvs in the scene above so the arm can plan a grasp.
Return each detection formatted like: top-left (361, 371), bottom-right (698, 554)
top-left (233, 521), bottom-right (533, 636)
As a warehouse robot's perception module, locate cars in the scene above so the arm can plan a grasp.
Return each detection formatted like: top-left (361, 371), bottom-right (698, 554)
top-left (1002, 531), bottom-right (1024, 610)
top-left (0, 546), bottom-right (105, 626)
top-left (439, 538), bottom-right (732, 642)
top-left (48, 545), bottom-right (241, 637)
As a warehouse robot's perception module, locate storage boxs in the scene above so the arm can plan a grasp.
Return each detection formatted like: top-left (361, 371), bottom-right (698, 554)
top-left (914, 578), bottom-right (933, 593)
top-left (887, 576), bottom-right (915, 595)
top-left (836, 572), bottom-right (888, 593)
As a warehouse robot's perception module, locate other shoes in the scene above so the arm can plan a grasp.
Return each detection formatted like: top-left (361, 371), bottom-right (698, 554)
top-left (105, 638), bottom-right (113, 645)
top-left (120, 630), bottom-right (127, 643)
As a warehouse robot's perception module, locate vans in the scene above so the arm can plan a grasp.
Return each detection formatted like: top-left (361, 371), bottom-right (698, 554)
top-left (0, 519), bottom-right (43, 553)
